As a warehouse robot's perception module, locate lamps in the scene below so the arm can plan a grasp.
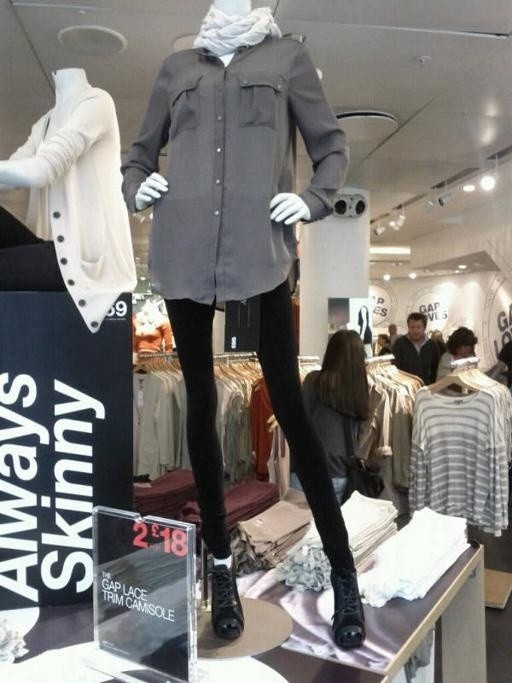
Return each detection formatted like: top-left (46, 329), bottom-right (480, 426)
top-left (370, 153), bottom-right (502, 236)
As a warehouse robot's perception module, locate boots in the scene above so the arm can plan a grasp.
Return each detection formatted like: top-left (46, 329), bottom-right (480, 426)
top-left (329, 569), bottom-right (366, 649)
top-left (206, 555), bottom-right (244, 640)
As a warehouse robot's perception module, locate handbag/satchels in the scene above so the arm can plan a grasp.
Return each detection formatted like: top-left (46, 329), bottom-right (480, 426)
top-left (346, 456), bottom-right (385, 499)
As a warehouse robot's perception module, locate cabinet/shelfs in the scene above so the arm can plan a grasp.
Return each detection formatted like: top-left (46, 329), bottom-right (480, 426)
top-left (1, 512), bottom-right (488, 683)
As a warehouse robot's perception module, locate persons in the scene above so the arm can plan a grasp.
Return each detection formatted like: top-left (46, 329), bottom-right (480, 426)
top-left (433, 325), bottom-right (478, 383)
top-left (490, 340), bottom-right (512, 393)
top-left (431, 328), bottom-right (445, 356)
top-left (391, 310), bottom-right (440, 384)
top-left (286, 327), bottom-right (373, 508)
top-left (120, 0), bottom-right (376, 651)
top-left (376, 334), bottom-right (391, 355)
top-left (355, 305), bottom-right (373, 343)
top-left (0, 63), bottom-right (142, 339)
top-left (130, 301), bottom-right (173, 366)
top-left (386, 322), bottom-right (400, 347)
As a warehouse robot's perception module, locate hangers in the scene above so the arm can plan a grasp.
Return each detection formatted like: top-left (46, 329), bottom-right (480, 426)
top-left (174, 353), bottom-right (265, 398)
top-left (363, 355), bottom-right (423, 401)
top-left (415, 368), bottom-right (510, 409)
top-left (250, 355), bottom-right (323, 386)
top-left (134, 352), bottom-right (185, 387)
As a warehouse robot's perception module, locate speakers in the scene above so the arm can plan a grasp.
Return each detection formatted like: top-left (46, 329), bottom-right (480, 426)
top-left (350, 194), bottom-right (369, 218)
top-left (333, 193), bottom-right (350, 218)
